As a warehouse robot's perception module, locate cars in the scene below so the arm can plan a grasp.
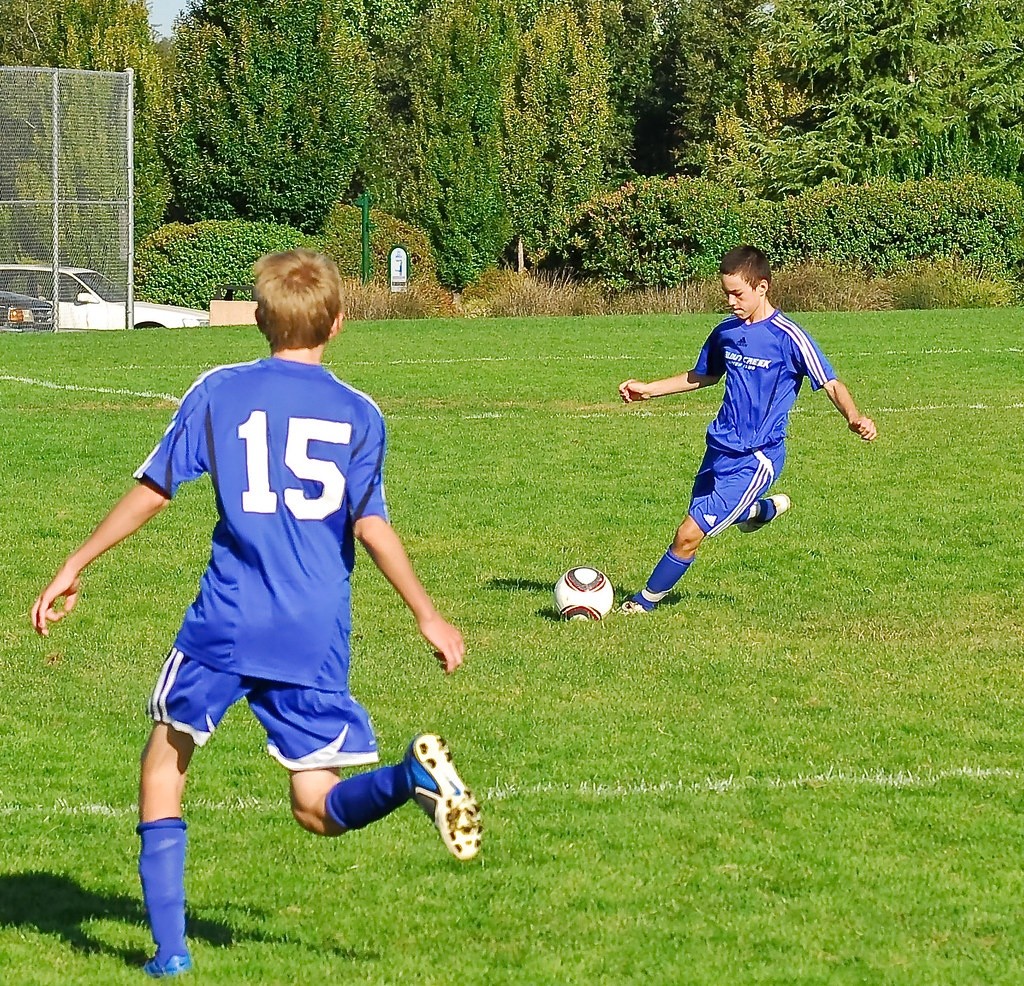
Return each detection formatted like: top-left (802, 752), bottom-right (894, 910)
top-left (0, 290), bottom-right (55, 331)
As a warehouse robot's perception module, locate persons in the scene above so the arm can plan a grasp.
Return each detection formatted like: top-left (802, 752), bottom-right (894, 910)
top-left (28, 249), bottom-right (483, 981)
top-left (619, 246), bottom-right (876, 614)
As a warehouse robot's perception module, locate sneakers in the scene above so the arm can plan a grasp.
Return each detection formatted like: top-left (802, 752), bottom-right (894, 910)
top-left (614, 602), bottom-right (648, 615)
top-left (737, 494), bottom-right (790, 534)
top-left (404, 733), bottom-right (482, 861)
top-left (127, 954), bottom-right (190, 978)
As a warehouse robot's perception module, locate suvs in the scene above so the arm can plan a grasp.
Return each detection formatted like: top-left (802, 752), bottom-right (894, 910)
top-left (0, 264), bottom-right (210, 329)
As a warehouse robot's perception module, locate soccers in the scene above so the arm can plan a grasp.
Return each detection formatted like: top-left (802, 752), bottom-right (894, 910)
top-left (554, 566), bottom-right (614, 623)
